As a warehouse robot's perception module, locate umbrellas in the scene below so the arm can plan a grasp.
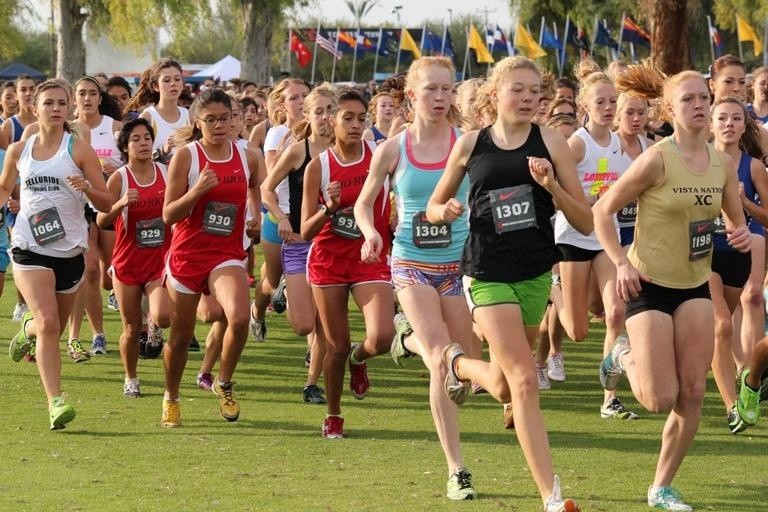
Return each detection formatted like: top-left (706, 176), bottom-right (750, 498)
top-left (0, 60), bottom-right (50, 79)
top-left (194, 53), bottom-right (242, 82)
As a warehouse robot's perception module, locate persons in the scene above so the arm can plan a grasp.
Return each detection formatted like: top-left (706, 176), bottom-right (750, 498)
top-left (617, 86), bottom-right (658, 250)
top-left (355, 55), bottom-right (478, 500)
top-left (2, 83), bottom-right (19, 123)
top-left (131, 56), bottom-right (193, 359)
top-left (299, 89), bottom-right (392, 439)
top-left (238, 98), bottom-right (258, 127)
top-left (425, 54), bottom-right (594, 510)
top-left (249, 121), bottom-right (283, 341)
top-left (696, 97), bottom-right (766, 434)
top-left (0, 73), bottom-right (48, 155)
top-left (591, 69), bottom-right (753, 510)
top-left (554, 57), bottom-right (636, 422)
top-left (707, 53), bottom-right (747, 106)
top-left (97, 118), bottom-right (172, 399)
top-left (158, 88), bottom-right (260, 426)
top-left (194, 95), bottom-right (263, 389)
top-left (538, 73), bottom-right (578, 136)
top-left (73, 74), bottom-right (126, 355)
top-left (745, 67), bottom-right (768, 131)
top-left (106, 75), bottom-right (138, 121)
top-left (362, 91), bottom-right (395, 139)
top-left (1, 80), bottom-right (111, 432)
top-left (259, 78), bottom-right (334, 403)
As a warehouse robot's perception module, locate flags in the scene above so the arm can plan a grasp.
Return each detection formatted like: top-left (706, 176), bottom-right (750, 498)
top-left (511, 16), bottom-right (547, 60)
top-left (706, 13), bottom-right (726, 65)
top-left (351, 26), bottom-right (376, 79)
top-left (591, 12), bottom-right (625, 57)
top-left (613, 9), bottom-right (652, 56)
top-left (488, 20), bottom-right (518, 64)
top-left (537, 14), bottom-right (562, 55)
top-left (394, 25), bottom-right (421, 75)
top-left (560, 13), bottom-right (592, 66)
top-left (288, 27), bottom-right (312, 74)
top-left (440, 24), bottom-right (457, 62)
top-left (484, 26), bottom-right (497, 47)
top-left (372, 23), bottom-right (401, 80)
top-left (734, 13), bottom-right (764, 64)
top-left (311, 21), bottom-right (345, 80)
top-left (460, 22), bottom-right (493, 78)
top-left (330, 26), bottom-right (356, 81)
top-left (419, 21), bottom-right (441, 55)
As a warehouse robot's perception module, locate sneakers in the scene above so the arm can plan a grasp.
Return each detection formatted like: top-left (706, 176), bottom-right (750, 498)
top-left (546, 352), bottom-right (566, 382)
top-left (472, 380), bottom-right (484, 394)
top-left (552, 274), bottom-right (562, 287)
top-left (247, 275), bottom-right (256, 287)
top-left (503, 403), bottom-right (515, 430)
top-left (737, 368), bottom-right (762, 426)
top-left (302, 385), bottom-right (327, 405)
top-left (440, 342), bottom-right (472, 405)
top-left (160, 398), bottom-right (181, 429)
top-left (645, 485), bottom-right (694, 511)
top-left (305, 350), bottom-right (311, 368)
top-left (195, 373), bottom-right (216, 390)
top-left (598, 333), bottom-right (631, 391)
top-left (138, 323), bottom-right (148, 359)
top-left (445, 465), bottom-right (478, 501)
top-left (66, 338), bottom-right (90, 363)
top-left (212, 376), bottom-right (240, 423)
top-left (48, 392), bottom-right (76, 429)
top-left (249, 302), bottom-right (268, 343)
top-left (107, 293), bottom-right (120, 312)
top-left (599, 398), bottom-right (639, 420)
top-left (23, 338), bottom-right (36, 362)
top-left (542, 474), bottom-right (580, 512)
top-left (321, 416), bottom-right (344, 439)
top-left (390, 312), bottom-right (417, 368)
top-left (12, 303), bottom-right (29, 322)
top-left (9, 310), bottom-right (34, 363)
top-left (90, 335), bottom-right (106, 354)
top-left (123, 381), bottom-right (141, 399)
top-left (535, 368), bottom-right (552, 390)
top-left (145, 313), bottom-right (164, 360)
top-left (188, 332), bottom-right (201, 351)
top-left (348, 344), bottom-right (371, 401)
top-left (727, 399), bottom-right (749, 434)
top-left (272, 279), bottom-right (287, 314)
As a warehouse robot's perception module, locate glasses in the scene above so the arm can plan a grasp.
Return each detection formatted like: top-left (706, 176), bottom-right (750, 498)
top-left (196, 115), bottom-right (234, 125)
